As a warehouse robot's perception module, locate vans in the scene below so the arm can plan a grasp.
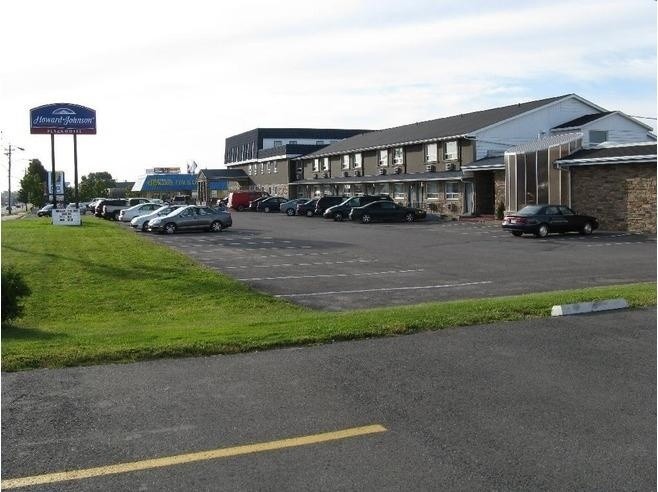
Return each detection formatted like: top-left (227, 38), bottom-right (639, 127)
top-left (322, 195), bottom-right (391, 222)
top-left (226, 191), bottom-right (271, 212)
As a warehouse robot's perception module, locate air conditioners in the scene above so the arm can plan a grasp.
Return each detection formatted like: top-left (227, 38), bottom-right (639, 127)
top-left (341, 167), bottom-right (400, 179)
top-left (445, 163), bottom-right (454, 171)
top-left (424, 165), bottom-right (434, 172)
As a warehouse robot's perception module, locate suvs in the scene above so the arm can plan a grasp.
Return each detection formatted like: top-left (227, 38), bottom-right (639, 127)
top-left (314, 195), bottom-right (356, 216)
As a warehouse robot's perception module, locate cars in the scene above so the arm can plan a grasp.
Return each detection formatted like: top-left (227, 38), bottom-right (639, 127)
top-left (37, 197), bottom-right (233, 235)
top-left (347, 200), bottom-right (428, 224)
top-left (500, 204), bottom-right (600, 239)
top-left (248, 196), bottom-right (319, 217)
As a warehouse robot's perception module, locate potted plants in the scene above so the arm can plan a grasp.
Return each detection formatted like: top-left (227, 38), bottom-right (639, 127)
top-left (495, 199), bottom-right (505, 220)
